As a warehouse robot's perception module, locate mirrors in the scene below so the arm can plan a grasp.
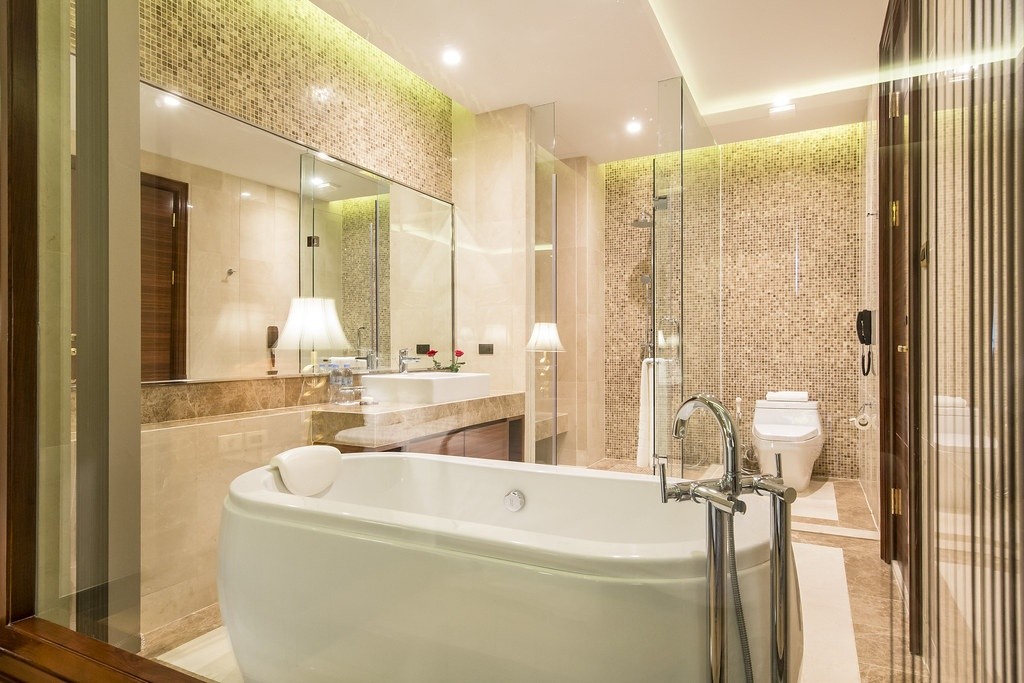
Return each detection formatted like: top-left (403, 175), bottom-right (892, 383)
top-left (137, 80), bottom-right (454, 386)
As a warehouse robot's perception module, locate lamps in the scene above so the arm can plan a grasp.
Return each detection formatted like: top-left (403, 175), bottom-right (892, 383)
top-left (526, 323), bottom-right (567, 362)
top-left (277, 298), bottom-right (352, 373)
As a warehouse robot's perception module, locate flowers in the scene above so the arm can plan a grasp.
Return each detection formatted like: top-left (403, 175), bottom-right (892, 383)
top-left (427, 350), bottom-right (442, 370)
top-left (449, 350), bottom-right (466, 372)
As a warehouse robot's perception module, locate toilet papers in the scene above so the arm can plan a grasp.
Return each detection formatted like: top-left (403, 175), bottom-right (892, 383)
top-left (766, 391), bottom-right (809, 402)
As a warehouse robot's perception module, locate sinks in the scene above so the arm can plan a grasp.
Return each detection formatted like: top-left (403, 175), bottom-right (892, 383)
top-left (361, 371), bottom-right (491, 404)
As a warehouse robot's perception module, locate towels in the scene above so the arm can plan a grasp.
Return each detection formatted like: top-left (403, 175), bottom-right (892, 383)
top-left (329, 356), bottom-right (368, 372)
top-left (636, 357), bottom-right (666, 469)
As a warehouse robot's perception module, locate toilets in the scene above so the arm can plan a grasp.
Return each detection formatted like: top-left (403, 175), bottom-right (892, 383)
top-left (750, 400), bottom-right (825, 493)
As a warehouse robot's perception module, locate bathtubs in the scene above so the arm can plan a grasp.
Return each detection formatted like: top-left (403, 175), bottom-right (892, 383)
top-left (219, 442), bottom-right (804, 683)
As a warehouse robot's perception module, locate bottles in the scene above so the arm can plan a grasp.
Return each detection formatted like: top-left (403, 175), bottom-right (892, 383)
top-left (330, 364), bottom-right (342, 404)
top-left (318, 364), bottom-right (332, 373)
top-left (342, 363), bottom-right (355, 403)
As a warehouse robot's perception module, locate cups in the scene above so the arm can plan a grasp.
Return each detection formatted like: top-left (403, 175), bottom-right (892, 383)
top-left (338, 385), bottom-right (366, 407)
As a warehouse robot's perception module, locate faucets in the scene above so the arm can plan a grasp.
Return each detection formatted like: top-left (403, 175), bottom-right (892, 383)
top-left (670, 391), bottom-right (742, 496)
top-left (355, 349), bottom-right (377, 373)
top-left (398, 348), bottom-right (422, 374)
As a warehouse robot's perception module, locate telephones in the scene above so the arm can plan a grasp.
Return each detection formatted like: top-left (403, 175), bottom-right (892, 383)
top-left (857, 309), bottom-right (871, 345)
